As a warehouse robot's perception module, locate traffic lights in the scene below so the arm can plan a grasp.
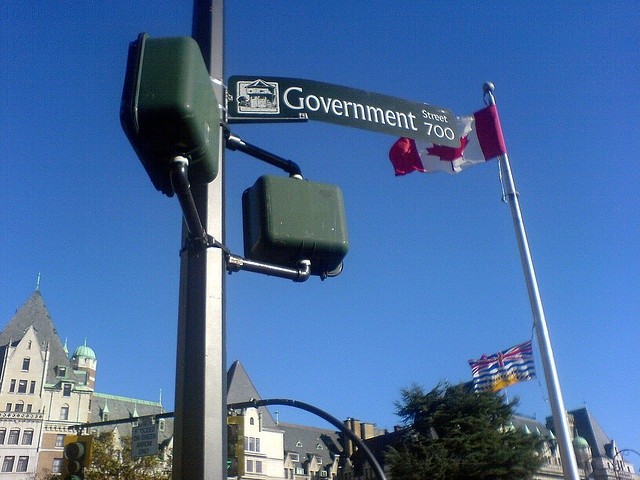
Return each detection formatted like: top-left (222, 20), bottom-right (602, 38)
top-left (227, 424), bottom-right (239, 477)
top-left (64, 442), bottom-right (85, 480)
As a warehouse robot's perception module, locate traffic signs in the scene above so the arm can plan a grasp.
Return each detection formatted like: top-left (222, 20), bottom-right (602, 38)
top-left (227, 75), bottom-right (461, 149)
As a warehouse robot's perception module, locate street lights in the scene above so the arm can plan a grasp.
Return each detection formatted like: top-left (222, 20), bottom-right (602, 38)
top-left (119, 0), bottom-right (349, 480)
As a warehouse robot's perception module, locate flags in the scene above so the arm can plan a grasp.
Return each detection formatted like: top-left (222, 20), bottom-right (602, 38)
top-left (389, 104), bottom-right (508, 177)
top-left (468, 340), bottom-right (538, 396)
